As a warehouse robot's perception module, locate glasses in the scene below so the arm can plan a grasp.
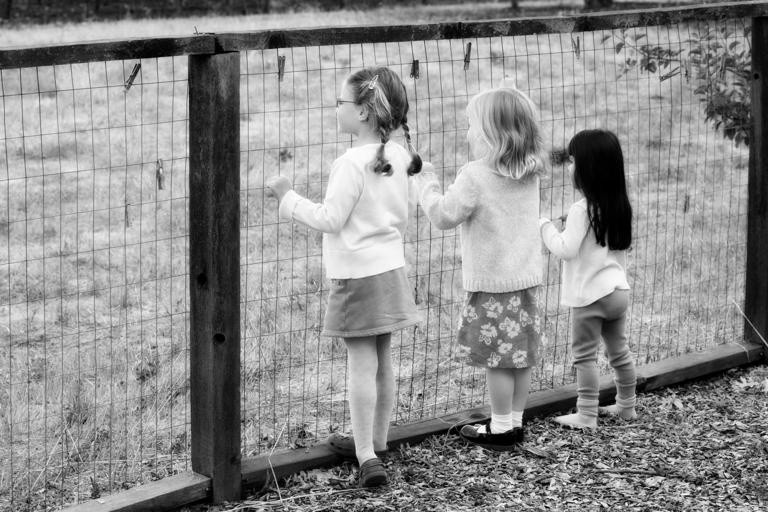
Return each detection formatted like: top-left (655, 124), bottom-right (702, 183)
top-left (335, 97), bottom-right (359, 107)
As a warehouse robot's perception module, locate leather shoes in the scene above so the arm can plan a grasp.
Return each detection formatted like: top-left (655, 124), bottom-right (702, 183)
top-left (328, 432), bottom-right (389, 485)
top-left (461, 422), bottom-right (525, 450)
